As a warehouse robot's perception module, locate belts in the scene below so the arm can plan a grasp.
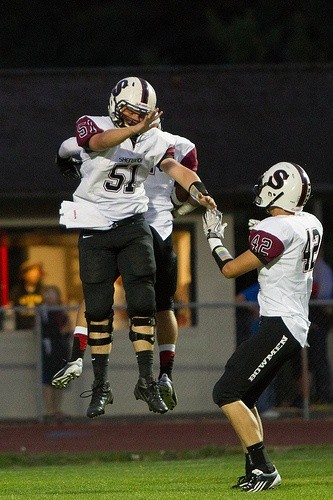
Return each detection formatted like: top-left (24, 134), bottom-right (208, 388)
top-left (81, 214), bottom-right (142, 233)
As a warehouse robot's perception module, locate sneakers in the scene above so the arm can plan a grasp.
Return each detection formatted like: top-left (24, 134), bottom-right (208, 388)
top-left (158, 373), bottom-right (178, 410)
top-left (51, 358), bottom-right (82, 389)
top-left (232, 471), bottom-right (256, 489)
top-left (87, 380), bottom-right (113, 418)
top-left (134, 377), bottom-right (168, 414)
top-left (241, 464), bottom-right (281, 493)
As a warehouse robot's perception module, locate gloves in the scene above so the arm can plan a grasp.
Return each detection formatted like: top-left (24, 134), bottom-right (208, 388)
top-left (248, 219), bottom-right (261, 230)
top-left (201, 207), bottom-right (228, 239)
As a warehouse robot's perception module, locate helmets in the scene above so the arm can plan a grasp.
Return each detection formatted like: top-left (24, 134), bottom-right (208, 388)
top-left (108, 77), bottom-right (157, 134)
top-left (251, 162), bottom-right (312, 212)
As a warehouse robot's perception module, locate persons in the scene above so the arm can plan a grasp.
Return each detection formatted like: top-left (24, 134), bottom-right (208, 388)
top-left (11, 245), bottom-right (332, 419)
top-left (202, 161), bottom-right (325, 495)
top-left (55, 113), bottom-right (207, 412)
top-left (55, 77), bottom-right (218, 419)
